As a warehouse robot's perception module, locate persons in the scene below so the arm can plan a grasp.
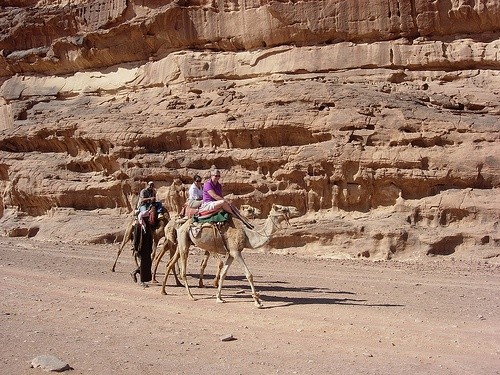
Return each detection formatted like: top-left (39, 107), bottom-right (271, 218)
top-left (131, 210), bottom-right (153, 287)
top-left (199, 169), bottom-right (238, 218)
top-left (137, 180), bottom-right (164, 220)
top-left (188, 174), bottom-right (203, 208)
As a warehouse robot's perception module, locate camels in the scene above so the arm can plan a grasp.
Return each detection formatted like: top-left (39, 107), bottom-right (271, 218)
top-left (160, 203), bottom-right (300, 309)
top-left (110, 205), bottom-right (262, 288)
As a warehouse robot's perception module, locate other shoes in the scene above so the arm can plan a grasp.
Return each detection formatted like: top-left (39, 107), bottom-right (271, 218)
top-left (130, 271), bottom-right (138, 283)
top-left (140, 282), bottom-right (149, 288)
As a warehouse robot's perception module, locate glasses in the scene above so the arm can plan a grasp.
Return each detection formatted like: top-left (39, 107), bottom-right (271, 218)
top-left (195, 180), bottom-right (202, 182)
top-left (211, 175), bottom-right (220, 177)
